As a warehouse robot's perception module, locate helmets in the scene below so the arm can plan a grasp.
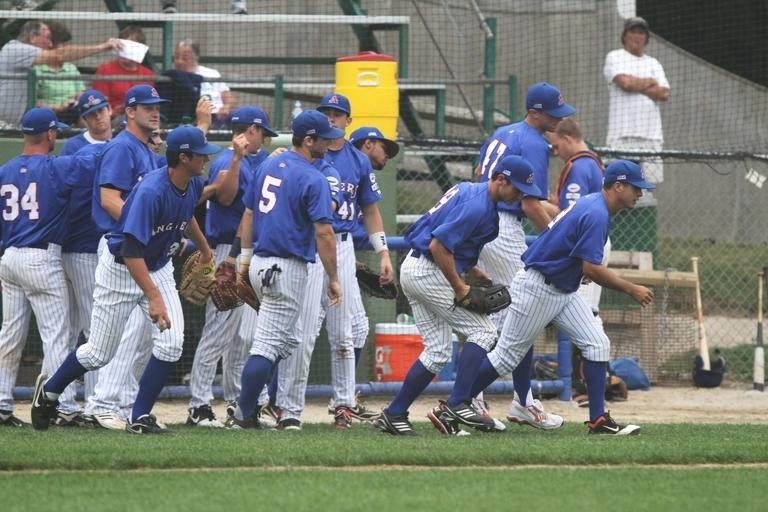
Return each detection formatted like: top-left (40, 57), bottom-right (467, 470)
top-left (692, 355), bottom-right (725, 386)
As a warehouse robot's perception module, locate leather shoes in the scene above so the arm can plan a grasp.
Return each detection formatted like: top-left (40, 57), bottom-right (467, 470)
top-left (162, 2), bottom-right (176, 13)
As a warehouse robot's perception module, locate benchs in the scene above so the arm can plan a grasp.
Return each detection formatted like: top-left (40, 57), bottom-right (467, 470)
top-left (0, 7), bottom-right (511, 317)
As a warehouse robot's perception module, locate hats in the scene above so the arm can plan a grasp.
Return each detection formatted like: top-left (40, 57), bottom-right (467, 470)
top-left (77, 90), bottom-right (109, 118)
top-left (291, 110), bottom-right (345, 140)
top-left (20, 106), bottom-right (69, 132)
top-left (316, 94), bottom-right (352, 117)
top-left (624, 16), bottom-right (649, 30)
top-left (166, 125), bottom-right (222, 156)
top-left (230, 106), bottom-right (279, 138)
top-left (495, 155), bottom-right (542, 196)
top-left (604, 159), bottom-right (657, 188)
top-left (526, 82), bottom-right (577, 118)
top-left (125, 83), bottom-right (171, 107)
top-left (349, 127), bottom-right (398, 159)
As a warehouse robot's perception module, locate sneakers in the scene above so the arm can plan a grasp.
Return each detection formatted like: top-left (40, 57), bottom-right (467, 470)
top-left (584, 413), bottom-right (640, 435)
top-left (427, 397), bottom-right (565, 436)
top-left (229, 0), bottom-right (247, 14)
top-left (328, 397), bottom-right (418, 436)
top-left (185, 400), bottom-right (302, 431)
top-left (0, 371), bottom-right (176, 433)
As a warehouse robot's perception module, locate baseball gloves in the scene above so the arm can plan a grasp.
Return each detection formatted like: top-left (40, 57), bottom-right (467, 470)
top-left (355, 267), bottom-right (397, 300)
top-left (454, 279), bottom-right (511, 315)
top-left (179, 249), bottom-right (260, 312)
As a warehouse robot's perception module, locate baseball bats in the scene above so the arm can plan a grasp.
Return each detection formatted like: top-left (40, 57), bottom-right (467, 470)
top-left (692, 256), bottom-right (711, 372)
top-left (753, 272), bottom-right (765, 390)
